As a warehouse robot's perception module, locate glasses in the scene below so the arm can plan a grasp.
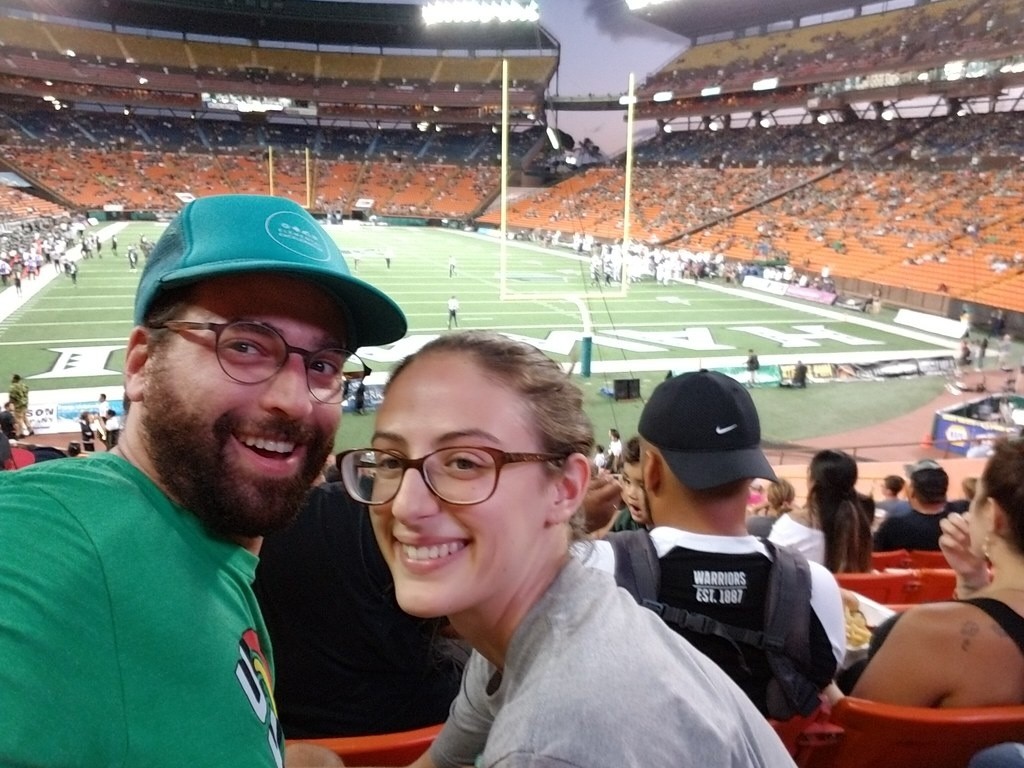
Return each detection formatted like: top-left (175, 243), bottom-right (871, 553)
top-left (335, 451), bottom-right (571, 506)
top-left (153, 320), bottom-right (375, 406)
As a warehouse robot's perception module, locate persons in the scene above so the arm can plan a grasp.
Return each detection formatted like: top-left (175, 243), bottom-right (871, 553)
top-left (337, 330), bottom-right (1024, 768)
top-left (0, 195), bottom-right (472, 768)
top-left (0, 113), bottom-right (1024, 334)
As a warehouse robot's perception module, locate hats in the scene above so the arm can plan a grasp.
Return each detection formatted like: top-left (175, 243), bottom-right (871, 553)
top-left (134, 193), bottom-right (408, 358)
top-left (638, 370), bottom-right (780, 491)
top-left (904, 461), bottom-right (948, 499)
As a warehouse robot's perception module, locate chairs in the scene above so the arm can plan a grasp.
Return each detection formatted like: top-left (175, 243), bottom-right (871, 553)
top-left (285, 548), bottom-right (1024, 768)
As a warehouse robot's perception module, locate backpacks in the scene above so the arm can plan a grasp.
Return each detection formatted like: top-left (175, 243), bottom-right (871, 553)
top-left (250, 481), bottom-right (449, 731)
